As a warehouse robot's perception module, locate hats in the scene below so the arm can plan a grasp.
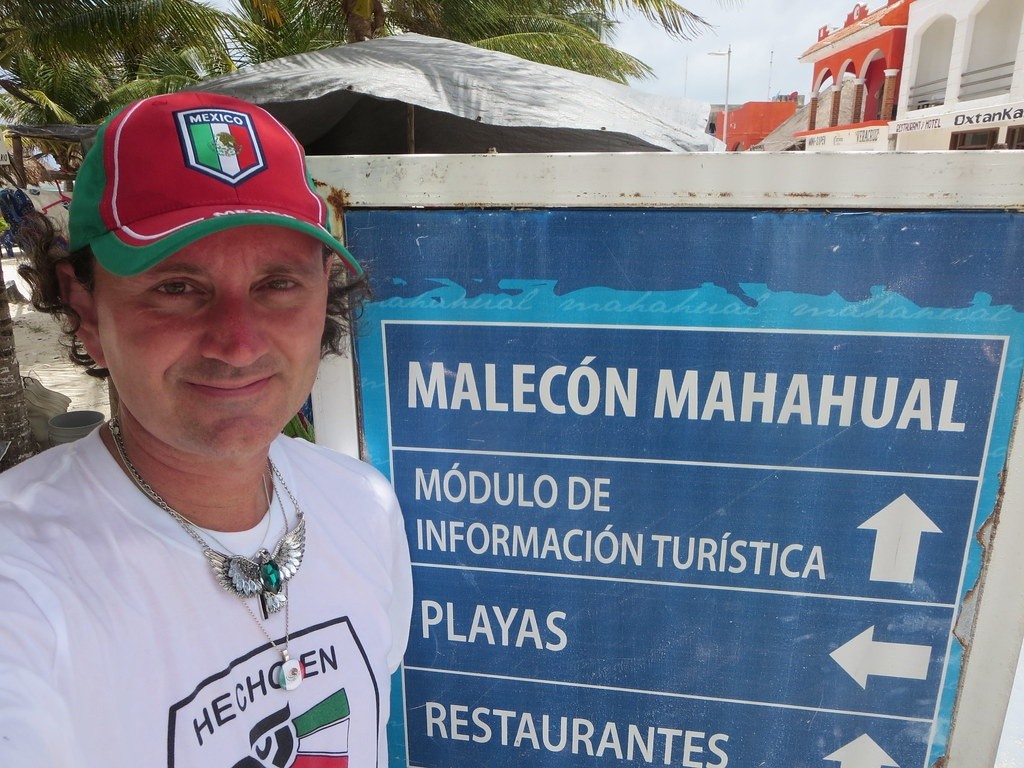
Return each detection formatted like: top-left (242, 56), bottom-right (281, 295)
top-left (64, 91), bottom-right (364, 281)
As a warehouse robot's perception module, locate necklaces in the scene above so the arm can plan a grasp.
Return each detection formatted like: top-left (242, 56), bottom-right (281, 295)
top-left (106, 416), bottom-right (307, 689)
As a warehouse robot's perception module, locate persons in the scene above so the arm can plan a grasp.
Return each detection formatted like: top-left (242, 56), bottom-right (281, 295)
top-left (0, 93), bottom-right (412, 768)
